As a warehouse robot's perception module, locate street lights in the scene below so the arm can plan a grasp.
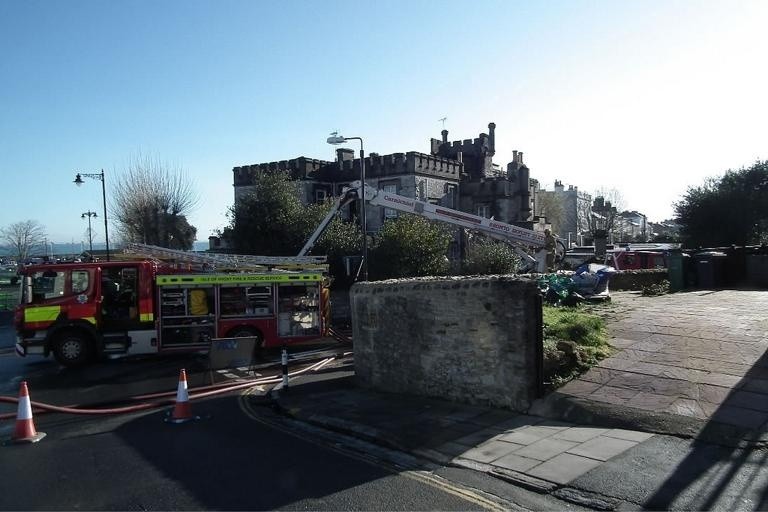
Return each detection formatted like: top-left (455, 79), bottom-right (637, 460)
top-left (325, 130), bottom-right (369, 282)
top-left (78, 210), bottom-right (98, 260)
top-left (71, 169), bottom-right (112, 262)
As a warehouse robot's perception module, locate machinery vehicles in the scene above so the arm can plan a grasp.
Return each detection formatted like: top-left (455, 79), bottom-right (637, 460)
top-left (293, 181), bottom-right (618, 303)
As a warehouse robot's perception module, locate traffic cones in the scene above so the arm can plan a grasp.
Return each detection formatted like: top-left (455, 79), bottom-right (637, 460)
top-left (161, 368), bottom-right (213, 425)
top-left (7, 381), bottom-right (48, 444)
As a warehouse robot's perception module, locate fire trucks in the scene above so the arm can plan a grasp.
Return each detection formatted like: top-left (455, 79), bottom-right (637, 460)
top-left (9, 241), bottom-right (344, 380)
